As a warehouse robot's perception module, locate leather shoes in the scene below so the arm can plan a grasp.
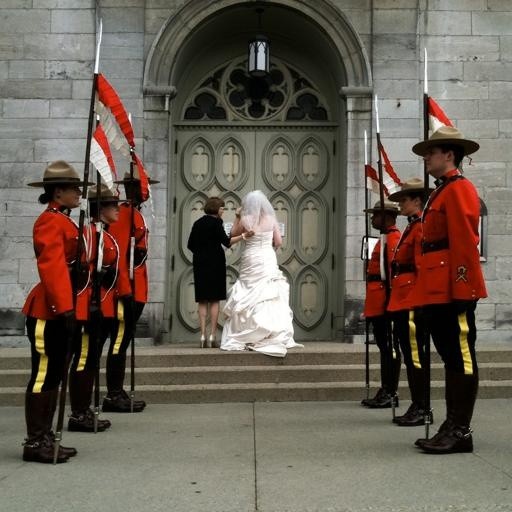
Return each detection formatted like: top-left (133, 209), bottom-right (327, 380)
top-left (393, 402), bottom-right (433, 426)
top-left (103, 388), bottom-right (146, 413)
top-left (68, 408), bottom-right (110, 432)
top-left (361, 389), bottom-right (401, 409)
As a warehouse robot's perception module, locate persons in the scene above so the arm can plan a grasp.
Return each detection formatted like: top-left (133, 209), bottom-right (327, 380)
top-left (67, 182), bottom-right (132, 434)
top-left (412, 126), bottom-right (490, 456)
top-left (219, 190), bottom-right (305, 360)
top-left (361, 197), bottom-right (407, 409)
top-left (386, 178), bottom-right (438, 428)
top-left (101, 167), bottom-right (160, 412)
top-left (187, 196), bottom-right (255, 349)
top-left (20, 160), bottom-right (95, 464)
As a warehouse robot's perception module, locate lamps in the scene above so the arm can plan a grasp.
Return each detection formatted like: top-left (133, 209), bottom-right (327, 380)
top-left (248, 4), bottom-right (271, 77)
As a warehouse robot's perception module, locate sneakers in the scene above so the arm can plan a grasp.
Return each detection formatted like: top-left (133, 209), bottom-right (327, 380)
top-left (415, 420), bottom-right (478, 454)
top-left (22, 440), bottom-right (76, 463)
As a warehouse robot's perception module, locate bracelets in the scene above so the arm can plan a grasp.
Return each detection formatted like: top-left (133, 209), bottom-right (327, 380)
top-left (241, 232), bottom-right (248, 241)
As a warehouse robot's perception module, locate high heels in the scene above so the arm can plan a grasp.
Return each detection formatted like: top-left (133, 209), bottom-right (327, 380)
top-left (200, 334), bottom-right (220, 348)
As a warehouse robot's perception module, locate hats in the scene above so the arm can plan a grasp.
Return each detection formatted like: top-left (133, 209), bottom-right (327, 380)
top-left (412, 126), bottom-right (479, 156)
top-left (27, 161), bottom-right (160, 204)
top-left (363, 178), bottom-right (435, 216)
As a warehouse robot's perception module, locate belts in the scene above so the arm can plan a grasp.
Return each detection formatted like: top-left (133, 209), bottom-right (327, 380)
top-left (366, 272), bottom-right (380, 281)
top-left (397, 263), bottom-right (416, 272)
top-left (421, 239), bottom-right (448, 254)
